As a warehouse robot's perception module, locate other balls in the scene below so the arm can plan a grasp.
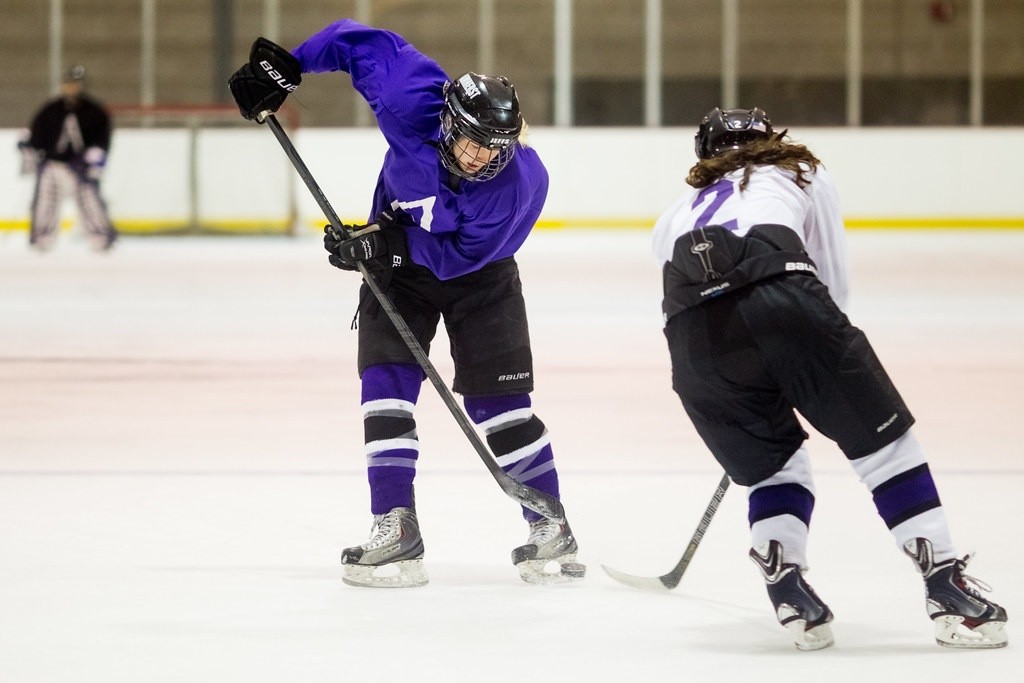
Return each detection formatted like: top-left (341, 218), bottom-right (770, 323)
top-left (561, 562), bottom-right (586, 578)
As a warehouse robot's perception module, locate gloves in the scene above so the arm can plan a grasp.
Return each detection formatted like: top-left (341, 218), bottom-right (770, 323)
top-left (324, 206), bottom-right (406, 273)
top-left (229, 38), bottom-right (299, 124)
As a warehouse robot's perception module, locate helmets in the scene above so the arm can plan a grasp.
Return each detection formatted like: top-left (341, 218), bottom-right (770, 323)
top-left (445, 71), bottom-right (525, 148)
top-left (60, 62), bottom-right (88, 84)
top-left (693, 105), bottom-right (777, 160)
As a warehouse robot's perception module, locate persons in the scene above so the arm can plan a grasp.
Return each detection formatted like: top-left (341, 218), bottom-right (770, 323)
top-left (229, 20), bottom-right (586, 586)
top-left (652, 108), bottom-right (1008, 651)
top-left (29, 64), bottom-right (117, 249)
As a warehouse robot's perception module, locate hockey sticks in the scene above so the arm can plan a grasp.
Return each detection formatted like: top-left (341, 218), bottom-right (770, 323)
top-left (256, 108), bottom-right (568, 526)
top-left (600, 472), bottom-right (733, 593)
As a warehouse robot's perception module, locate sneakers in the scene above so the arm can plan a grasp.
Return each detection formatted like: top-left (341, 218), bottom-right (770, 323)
top-left (748, 537), bottom-right (836, 650)
top-left (337, 508), bottom-right (431, 588)
top-left (510, 516), bottom-right (585, 583)
top-left (919, 559), bottom-right (1012, 648)
